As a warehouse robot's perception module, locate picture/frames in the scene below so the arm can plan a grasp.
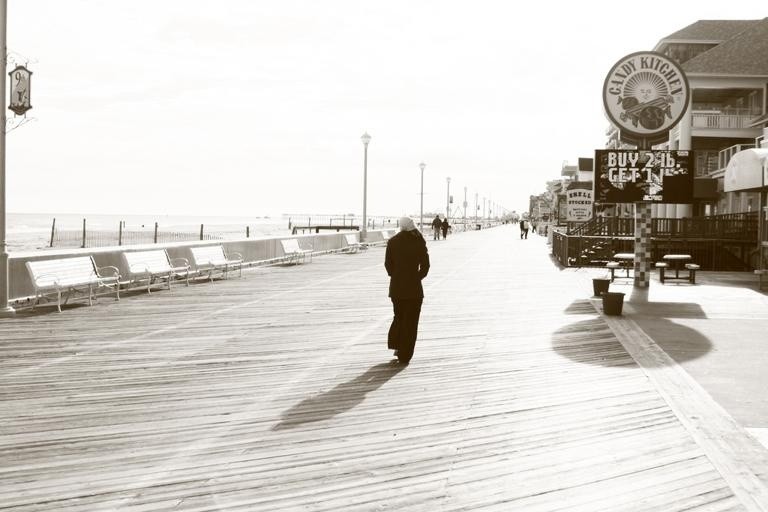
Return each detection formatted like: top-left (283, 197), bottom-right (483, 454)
top-left (614, 253), bottom-right (635, 278)
top-left (663, 254), bottom-right (692, 280)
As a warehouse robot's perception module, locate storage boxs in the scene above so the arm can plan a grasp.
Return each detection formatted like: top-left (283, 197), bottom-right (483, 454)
top-left (381, 232), bottom-right (391, 244)
top-left (345, 234), bottom-right (368, 254)
top-left (190, 245), bottom-right (244, 283)
top-left (25, 255), bottom-right (122, 313)
top-left (122, 248), bottom-right (192, 295)
top-left (280, 239), bottom-right (314, 266)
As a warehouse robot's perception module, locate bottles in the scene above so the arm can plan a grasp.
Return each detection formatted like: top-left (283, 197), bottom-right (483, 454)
top-left (400, 217), bottom-right (416, 231)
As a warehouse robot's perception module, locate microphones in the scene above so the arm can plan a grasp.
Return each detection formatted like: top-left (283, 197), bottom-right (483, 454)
top-left (655, 263), bottom-right (670, 283)
top-left (685, 264), bottom-right (700, 283)
top-left (606, 262), bottom-right (619, 282)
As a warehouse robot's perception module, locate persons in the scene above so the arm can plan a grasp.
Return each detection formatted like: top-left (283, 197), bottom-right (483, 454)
top-left (520, 220), bottom-right (537, 239)
top-left (432, 215), bottom-right (442, 240)
top-left (442, 217), bottom-right (451, 240)
top-left (384, 214), bottom-right (431, 362)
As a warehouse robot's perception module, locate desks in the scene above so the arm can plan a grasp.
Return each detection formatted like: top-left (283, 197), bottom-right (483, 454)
top-left (418, 163), bottom-right (426, 236)
top-left (482, 197), bottom-right (518, 229)
top-left (446, 177), bottom-right (451, 229)
top-left (475, 193), bottom-right (478, 229)
top-left (360, 132), bottom-right (372, 249)
top-left (462, 186), bottom-right (467, 232)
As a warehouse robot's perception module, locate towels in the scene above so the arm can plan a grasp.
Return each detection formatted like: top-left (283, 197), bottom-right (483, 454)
top-left (593, 278), bottom-right (610, 296)
top-left (602, 292), bottom-right (626, 315)
